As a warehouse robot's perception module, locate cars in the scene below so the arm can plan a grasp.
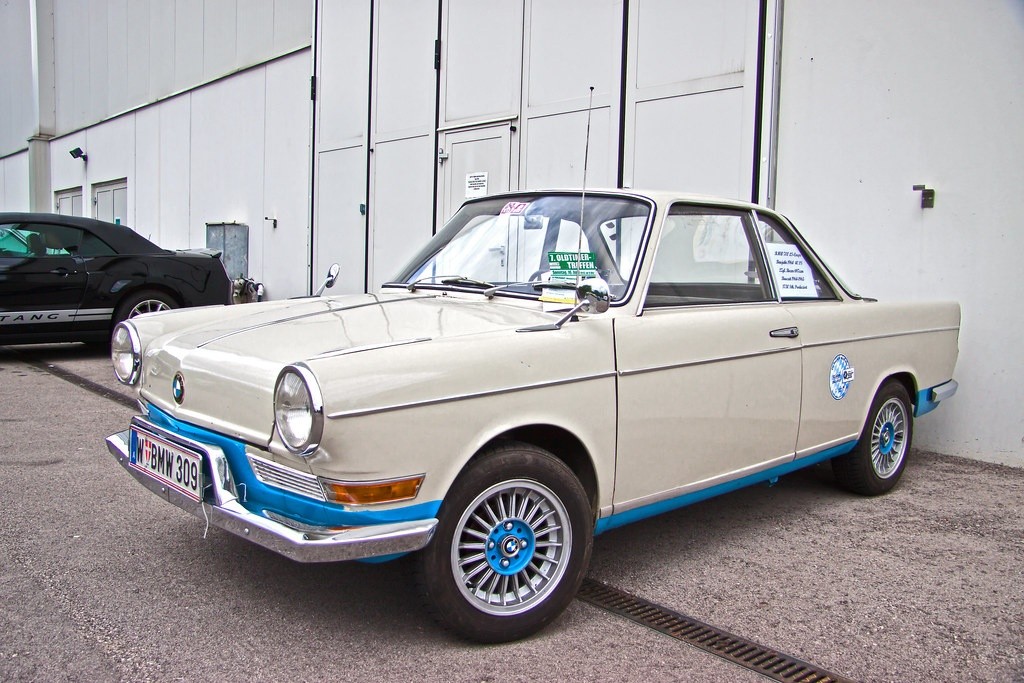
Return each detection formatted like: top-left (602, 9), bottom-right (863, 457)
top-left (106, 84), bottom-right (963, 646)
top-left (0, 209), bottom-right (232, 351)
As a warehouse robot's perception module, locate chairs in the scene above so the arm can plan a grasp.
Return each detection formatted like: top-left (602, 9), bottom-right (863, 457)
top-left (39, 230), bottom-right (63, 254)
